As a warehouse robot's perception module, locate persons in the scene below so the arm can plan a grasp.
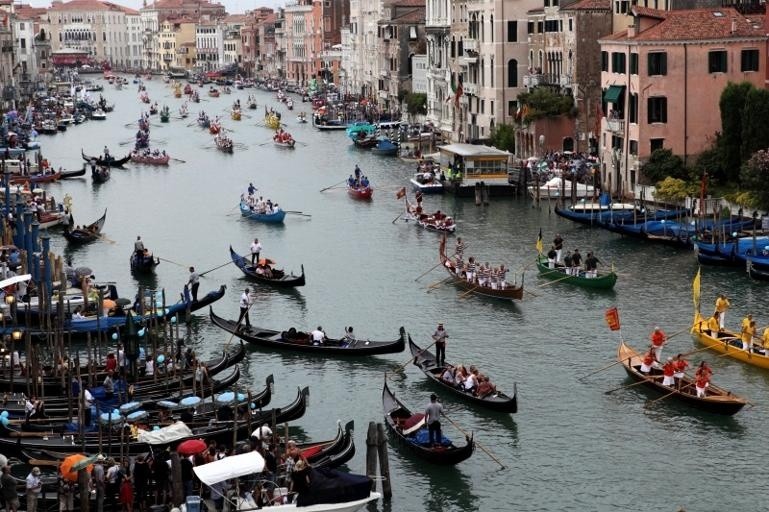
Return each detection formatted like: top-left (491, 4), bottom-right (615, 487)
top-left (2, 62), bottom-right (371, 511)
top-left (431, 322), bottom-right (450, 368)
top-left (439, 361), bottom-right (497, 398)
top-left (519, 148), bottom-right (596, 182)
top-left (451, 236), bottom-right (509, 290)
top-left (638, 294), bottom-right (769, 403)
top-left (382, 117), bottom-right (447, 187)
top-left (409, 190), bottom-right (452, 228)
top-left (547, 233), bottom-right (604, 280)
top-left (426, 393), bottom-right (444, 447)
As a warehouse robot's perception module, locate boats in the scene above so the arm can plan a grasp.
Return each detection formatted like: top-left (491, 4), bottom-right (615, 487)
top-left (554, 206), bottom-right (769, 276)
top-left (240, 200), bottom-right (285, 223)
top-left (2, 187), bottom-right (108, 245)
top-left (81, 148), bottom-right (169, 183)
top-left (618, 341), bottom-right (747, 416)
top-left (440, 253), bottom-right (523, 300)
top-left (312, 106), bottom-right (345, 131)
top-left (12, 163), bottom-right (86, 184)
top-left (230, 246), bottom-right (305, 286)
top-left (408, 334), bottom-right (517, 413)
top-left (536, 253), bottom-right (617, 288)
top-left (349, 121), bottom-right (379, 144)
top-left (210, 306), bottom-right (405, 355)
top-left (133, 74), bottom-right (307, 153)
top-left (35, 70), bottom-right (128, 134)
top-left (131, 250), bottom-right (160, 271)
top-left (405, 200), bottom-right (457, 234)
top-left (346, 180), bottom-right (373, 200)
top-left (528, 176), bottom-right (600, 199)
top-left (382, 372), bottom-right (473, 465)
top-left (410, 178), bottom-right (443, 193)
top-left (694, 310), bottom-right (769, 369)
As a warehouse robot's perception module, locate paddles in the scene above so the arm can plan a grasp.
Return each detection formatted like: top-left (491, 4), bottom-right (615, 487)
top-left (321, 179), bottom-right (593, 301)
top-left (447, 416), bottom-right (504, 468)
top-left (394, 336), bottom-right (445, 374)
top-left (119, 113), bottom-right (287, 163)
top-left (89, 189), bottom-right (312, 351)
top-left (578, 302), bottom-right (767, 408)
